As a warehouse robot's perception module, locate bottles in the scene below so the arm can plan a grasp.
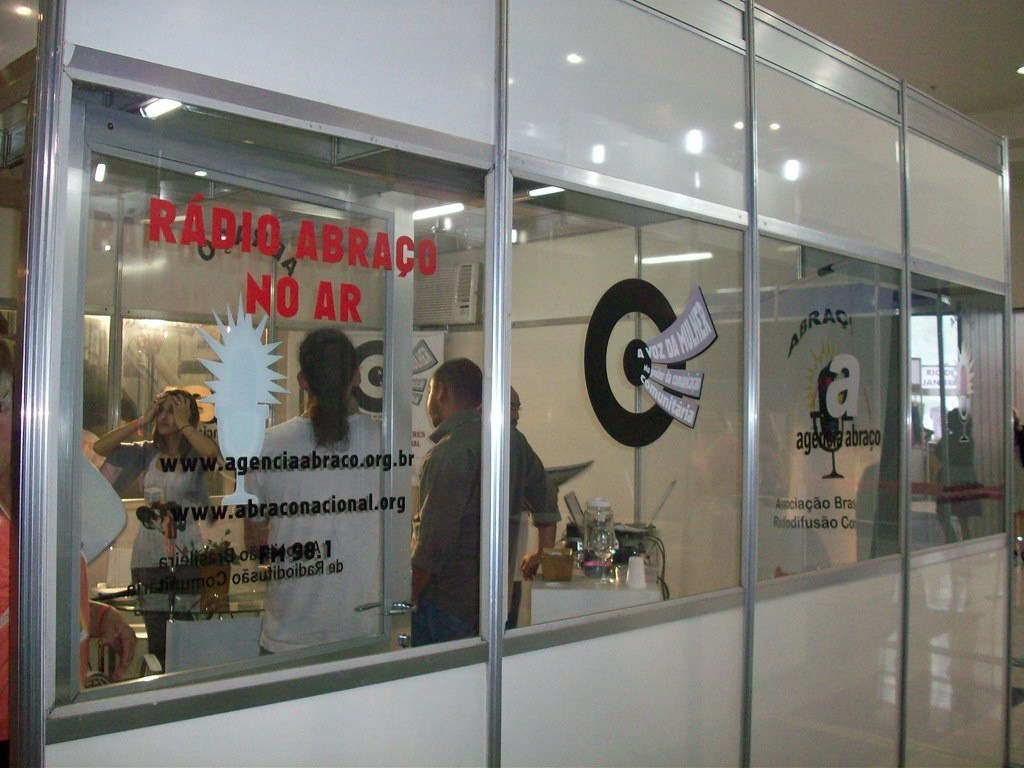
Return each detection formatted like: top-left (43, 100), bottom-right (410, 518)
top-left (539, 499), bottom-right (649, 587)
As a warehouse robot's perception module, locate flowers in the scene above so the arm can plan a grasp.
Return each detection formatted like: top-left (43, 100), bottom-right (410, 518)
top-left (191, 530), bottom-right (242, 567)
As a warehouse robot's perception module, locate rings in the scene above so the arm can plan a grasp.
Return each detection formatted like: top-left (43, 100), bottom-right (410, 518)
top-left (177, 402), bottom-right (180, 404)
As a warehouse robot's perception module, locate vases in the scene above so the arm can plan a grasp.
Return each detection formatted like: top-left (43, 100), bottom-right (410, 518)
top-left (200, 565), bottom-right (231, 612)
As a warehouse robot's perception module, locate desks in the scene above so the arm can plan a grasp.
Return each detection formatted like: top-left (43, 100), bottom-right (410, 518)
top-left (99, 592), bottom-right (266, 678)
top-left (531, 564), bottom-right (659, 626)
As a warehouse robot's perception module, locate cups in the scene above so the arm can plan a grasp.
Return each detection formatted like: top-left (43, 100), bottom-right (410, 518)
top-left (242, 518), bottom-right (270, 559)
top-left (200, 564), bottom-right (230, 613)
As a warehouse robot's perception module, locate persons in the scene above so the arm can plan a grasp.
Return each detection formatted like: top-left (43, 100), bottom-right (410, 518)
top-left (92, 387), bottom-right (221, 675)
top-left (505, 386), bottom-right (528, 630)
top-left (0, 311), bottom-right (138, 768)
top-left (932, 407), bottom-right (983, 542)
top-left (242, 328), bottom-right (419, 656)
top-left (411, 358), bottom-right (560, 648)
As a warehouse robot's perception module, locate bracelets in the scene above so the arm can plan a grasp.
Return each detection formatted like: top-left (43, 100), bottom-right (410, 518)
top-left (180, 425), bottom-right (192, 431)
top-left (96, 604), bottom-right (110, 632)
